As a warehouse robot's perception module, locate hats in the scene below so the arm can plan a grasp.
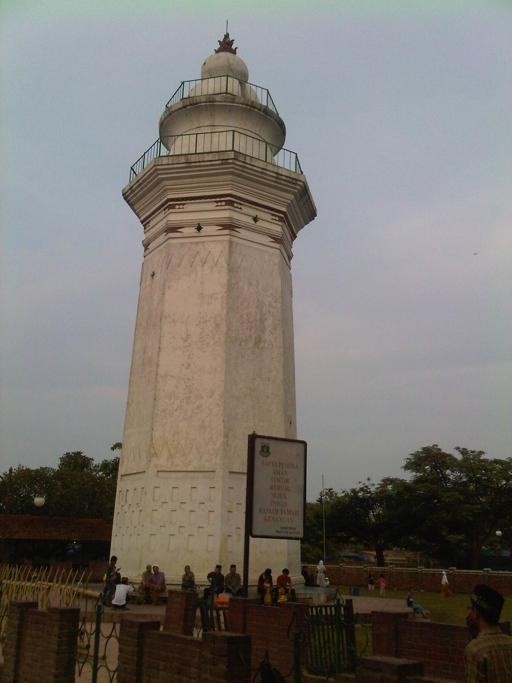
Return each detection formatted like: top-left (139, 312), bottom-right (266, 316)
top-left (468, 584), bottom-right (505, 618)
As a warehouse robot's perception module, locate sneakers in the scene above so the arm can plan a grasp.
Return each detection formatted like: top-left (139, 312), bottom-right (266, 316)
top-left (112, 606), bottom-right (130, 610)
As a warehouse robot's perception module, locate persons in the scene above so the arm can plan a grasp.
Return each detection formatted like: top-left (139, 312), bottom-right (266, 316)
top-left (375, 573), bottom-right (386, 598)
top-left (366, 572), bottom-right (376, 594)
top-left (461, 583), bottom-right (512, 683)
top-left (407, 586), bottom-right (429, 619)
top-left (316, 560), bottom-right (327, 586)
top-left (181, 565), bottom-right (195, 591)
top-left (204, 565), bottom-right (224, 600)
top-left (256, 568), bottom-right (297, 607)
top-left (440, 571), bottom-right (455, 598)
top-left (100, 555), bottom-right (167, 610)
top-left (224, 564), bottom-right (242, 597)
top-left (301, 565), bottom-right (310, 586)
